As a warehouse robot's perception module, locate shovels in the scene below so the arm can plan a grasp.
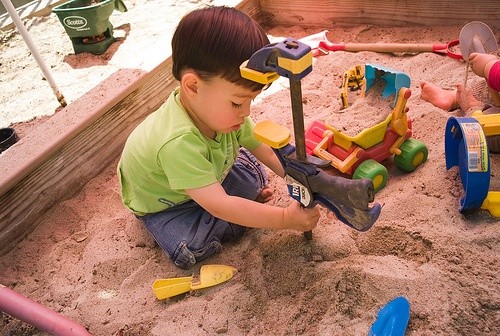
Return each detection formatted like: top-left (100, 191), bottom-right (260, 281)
top-left (294, 31), bottom-right (464, 61)
top-left (152, 264), bottom-right (238, 302)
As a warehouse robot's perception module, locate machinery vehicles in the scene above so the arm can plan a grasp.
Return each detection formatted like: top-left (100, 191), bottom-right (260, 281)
top-left (302, 63), bottom-right (428, 195)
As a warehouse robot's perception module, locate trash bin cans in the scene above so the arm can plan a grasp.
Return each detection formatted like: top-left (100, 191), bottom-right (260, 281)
top-left (50, 0), bottom-right (128, 56)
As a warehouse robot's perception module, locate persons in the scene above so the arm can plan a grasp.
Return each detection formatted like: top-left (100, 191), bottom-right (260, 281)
top-left (117, 4), bottom-right (320, 270)
top-left (419, 52), bottom-right (500, 154)
